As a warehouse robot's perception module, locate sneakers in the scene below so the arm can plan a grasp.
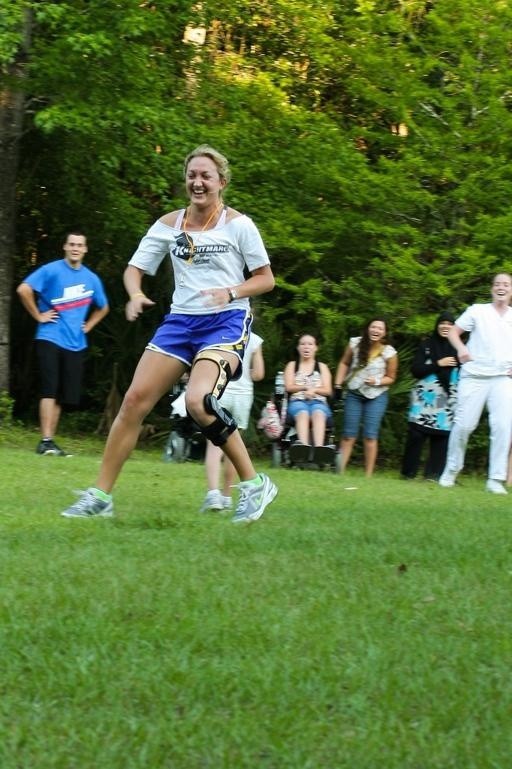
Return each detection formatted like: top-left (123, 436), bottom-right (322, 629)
top-left (55, 485), bottom-right (116, 520)
top-left (230, 472), bottom-right (280, 526)
top-left (436, 464), bottom-right (461, 487)
top-left (484, 477), bottom-right (507, 495)
top-left (199, 491), bottom-right (234, 513)
top-left (36, 437), bottom-right (74, 458)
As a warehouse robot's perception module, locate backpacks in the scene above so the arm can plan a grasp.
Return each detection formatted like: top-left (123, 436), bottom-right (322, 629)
top-left (257, 398), bottom-right (289, 440)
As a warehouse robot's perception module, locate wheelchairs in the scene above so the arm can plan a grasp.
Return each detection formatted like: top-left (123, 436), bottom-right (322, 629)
top-left (167, 370), bottom-right (207, 463)
top-left (272, 371), bottom-right (341, 474)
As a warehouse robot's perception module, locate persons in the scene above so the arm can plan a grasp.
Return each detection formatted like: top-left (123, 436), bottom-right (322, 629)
top-left (59, 144), bottom-right (278, 526)
top-left (16, 233), bottom-right (110, 457)
